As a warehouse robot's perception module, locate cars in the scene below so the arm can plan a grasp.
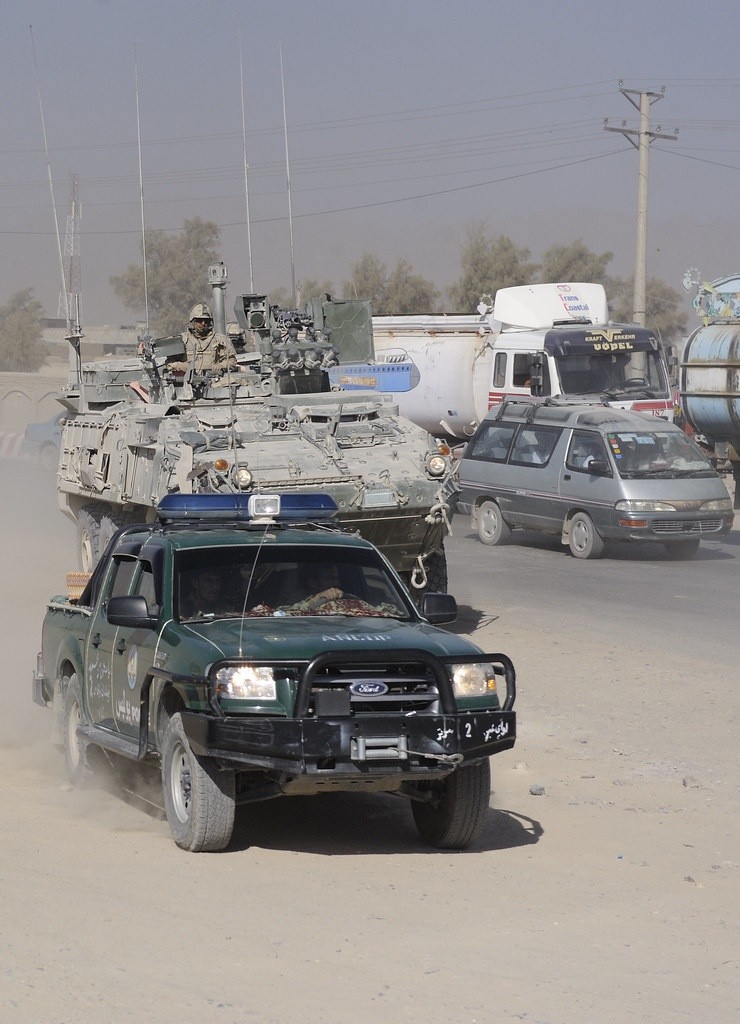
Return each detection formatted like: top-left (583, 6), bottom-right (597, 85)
top-left (30, 491), bottom-right (517, 855)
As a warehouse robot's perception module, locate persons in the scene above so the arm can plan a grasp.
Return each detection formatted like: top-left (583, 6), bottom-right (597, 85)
top-left (164, 301), bottom-right (239, 373)
top-left (271, 551), bottom-right (346, 615)
top-left (588, 353), bottom-right (632, 392)
top-left (175, 557), bottom-right (250, 620)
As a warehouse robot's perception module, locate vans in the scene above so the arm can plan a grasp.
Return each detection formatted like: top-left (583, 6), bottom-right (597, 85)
top-left (452, 393), bottom-right (734, 560)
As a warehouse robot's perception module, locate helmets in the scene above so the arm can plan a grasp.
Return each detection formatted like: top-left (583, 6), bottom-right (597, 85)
top-left (189, 302), bottom-right (213, 322)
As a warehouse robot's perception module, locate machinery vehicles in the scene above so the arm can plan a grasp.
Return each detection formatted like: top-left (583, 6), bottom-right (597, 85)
top-left (57, 263), bottom-right (456, 628)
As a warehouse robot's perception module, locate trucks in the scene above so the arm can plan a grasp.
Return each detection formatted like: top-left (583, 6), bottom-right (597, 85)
top-left (372, 280), bottom-right (676, 443)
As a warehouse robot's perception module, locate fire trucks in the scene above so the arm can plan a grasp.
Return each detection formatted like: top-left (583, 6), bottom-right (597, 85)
top-left (679, 272), bottom-right (740, 449)
